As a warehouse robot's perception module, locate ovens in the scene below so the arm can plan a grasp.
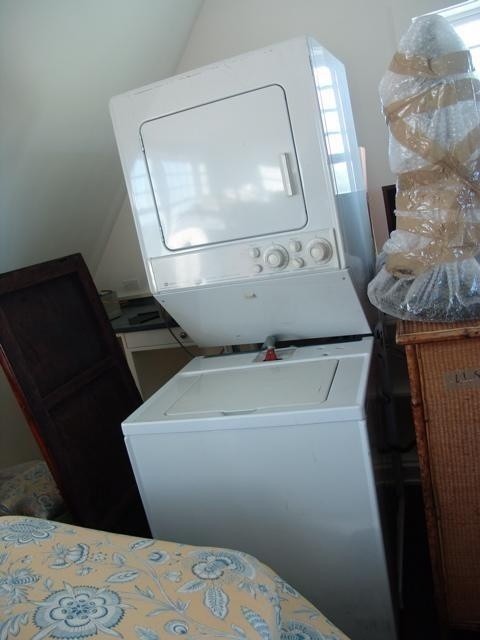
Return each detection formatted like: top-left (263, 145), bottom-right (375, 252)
top-left (108, 33), bottom-right (376, 295)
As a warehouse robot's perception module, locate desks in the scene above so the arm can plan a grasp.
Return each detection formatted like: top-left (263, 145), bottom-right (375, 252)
top-left (109, 296), bottom-right (239, 402)
top-left (1, 460), bottom-right (63, 525)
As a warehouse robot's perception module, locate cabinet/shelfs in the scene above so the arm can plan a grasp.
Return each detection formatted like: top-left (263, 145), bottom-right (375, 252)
top-left (394, 316), bottom-right (480, 640)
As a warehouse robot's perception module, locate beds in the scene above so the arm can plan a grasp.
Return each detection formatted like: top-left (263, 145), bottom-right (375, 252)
top-left (1, 514), bottom-right (345, 640)
